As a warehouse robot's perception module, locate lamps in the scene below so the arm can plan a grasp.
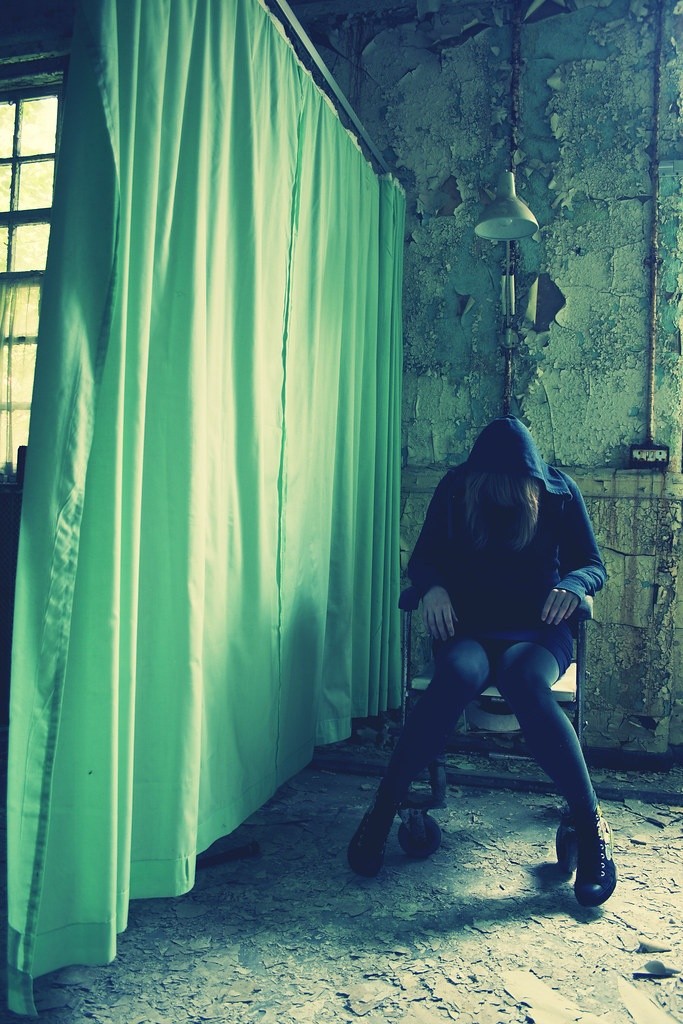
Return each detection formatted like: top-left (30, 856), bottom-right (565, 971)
top-left (472, 168), bottom-right (542, 349)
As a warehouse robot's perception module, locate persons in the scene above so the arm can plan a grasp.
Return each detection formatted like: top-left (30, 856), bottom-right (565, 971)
top-left (345, 415), bottom-right (616, 907)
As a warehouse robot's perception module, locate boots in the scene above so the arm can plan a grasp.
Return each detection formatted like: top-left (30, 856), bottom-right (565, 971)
top-left (574, 806), bottom-right (617, 907)
top-left (347, 791), bottom-right (397, 874)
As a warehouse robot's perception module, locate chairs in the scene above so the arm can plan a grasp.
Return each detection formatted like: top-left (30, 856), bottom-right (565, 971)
top-left (393, 583), bottom-right (595, 876)
